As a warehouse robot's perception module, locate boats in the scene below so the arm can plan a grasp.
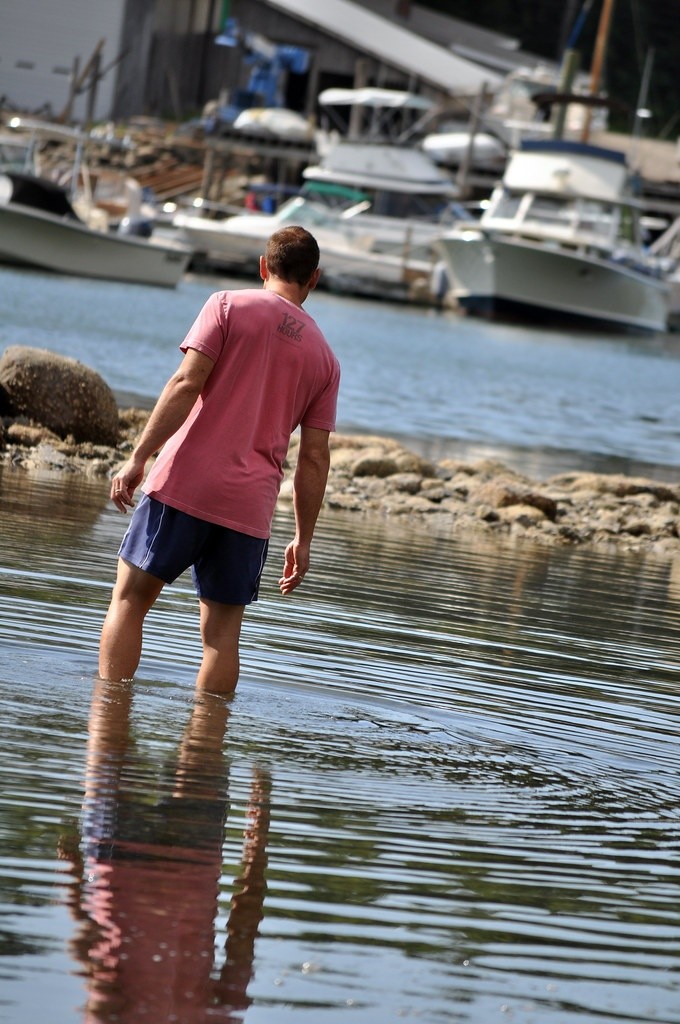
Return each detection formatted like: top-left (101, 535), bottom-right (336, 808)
top-left (171, 87), bottom-right (470, 307)
top-left (0, 115), bottom-right (197, 290)
top-left (433, 136), bottom-right (671, 337)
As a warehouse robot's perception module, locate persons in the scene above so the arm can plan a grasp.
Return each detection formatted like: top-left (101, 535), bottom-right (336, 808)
top-left (98, 225), bottom-right (343, 691)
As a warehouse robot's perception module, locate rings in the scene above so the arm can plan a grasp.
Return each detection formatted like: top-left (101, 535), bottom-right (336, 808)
top-left (299, 575), bottom-right (303, 579)
top-left (115, 489), bottom-right (120, 492)
